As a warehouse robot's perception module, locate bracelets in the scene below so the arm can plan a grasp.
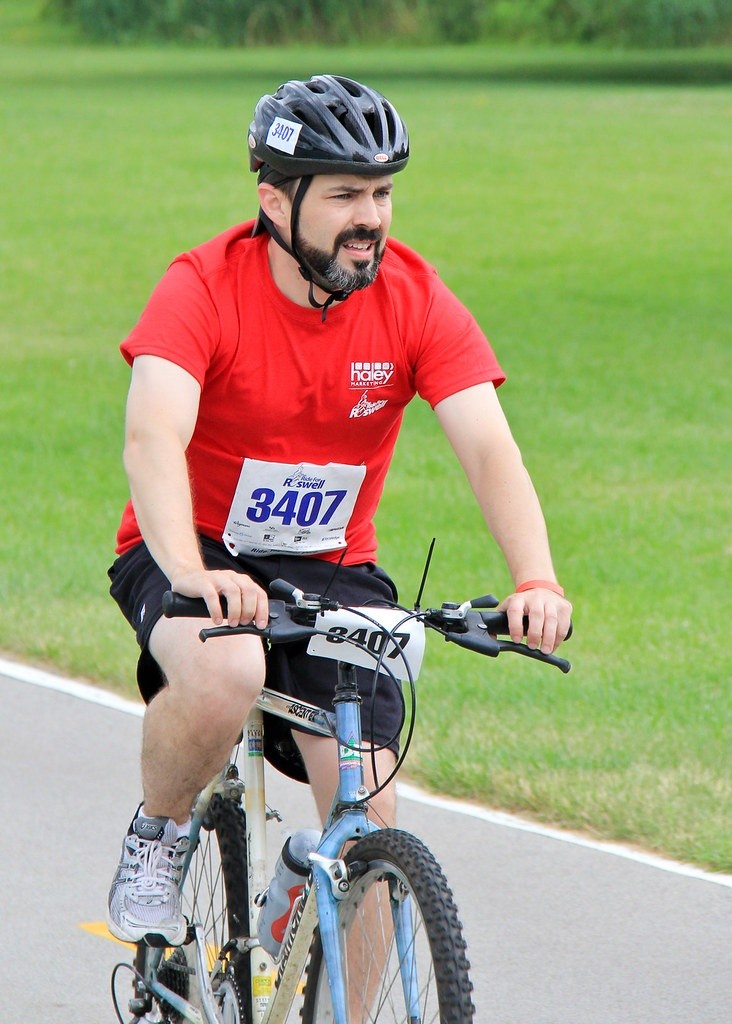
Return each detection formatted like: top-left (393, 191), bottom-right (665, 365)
top-left (515, 579), bottom-right (566, 598)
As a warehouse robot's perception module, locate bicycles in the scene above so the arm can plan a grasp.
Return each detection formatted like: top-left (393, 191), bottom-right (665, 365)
top-left (109, 575), bottom-right (573, 1023)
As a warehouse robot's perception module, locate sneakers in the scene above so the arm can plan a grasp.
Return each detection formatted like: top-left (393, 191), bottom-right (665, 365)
top-left (107, 804), bottom-right (198, 948)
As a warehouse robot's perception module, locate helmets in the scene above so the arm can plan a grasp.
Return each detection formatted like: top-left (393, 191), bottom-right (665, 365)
top-left (247, 73), bottom-right (411, 176)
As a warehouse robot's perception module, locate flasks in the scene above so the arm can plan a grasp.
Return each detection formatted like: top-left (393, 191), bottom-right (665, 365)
top-left (256, 829), bottom-right (323, 959)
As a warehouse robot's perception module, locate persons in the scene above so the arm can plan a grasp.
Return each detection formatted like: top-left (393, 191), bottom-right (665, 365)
top-left (102, 69), bottom-right (574, 1024)
